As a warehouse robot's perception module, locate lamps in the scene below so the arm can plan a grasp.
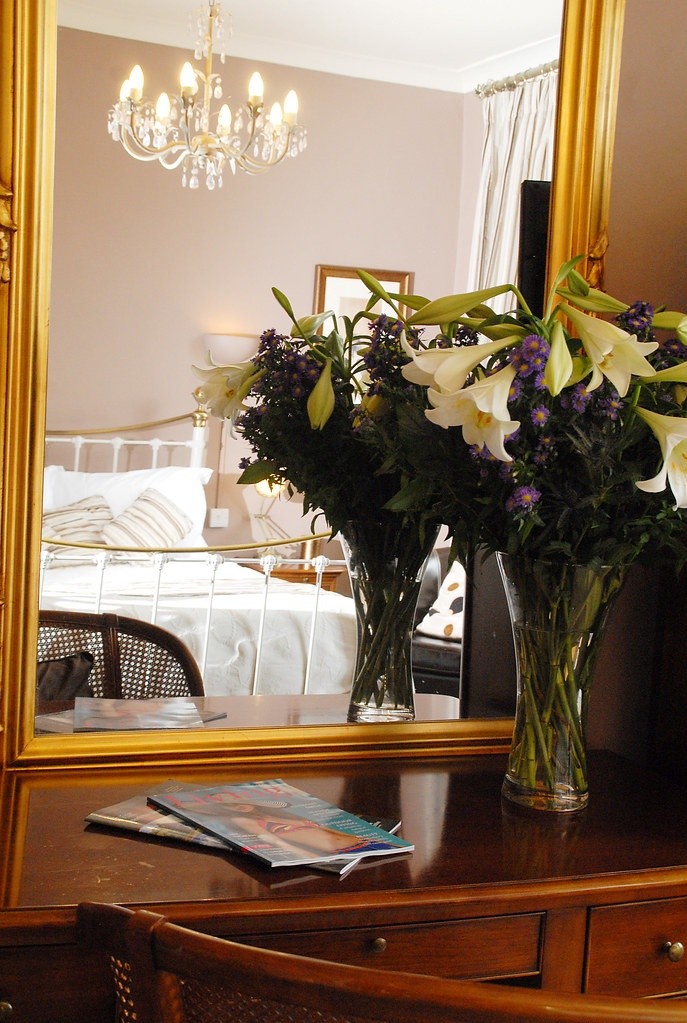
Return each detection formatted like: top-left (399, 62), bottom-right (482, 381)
top-left (108, 1), bottom-right (305, 189)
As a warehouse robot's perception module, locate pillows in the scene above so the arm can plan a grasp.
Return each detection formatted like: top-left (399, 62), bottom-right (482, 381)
top-left (42, 495), bottom-right (115, 568)
top-left (102, 487), bottom-right (194, 565)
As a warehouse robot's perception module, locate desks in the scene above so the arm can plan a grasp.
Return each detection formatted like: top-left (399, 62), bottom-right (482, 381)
top-left (0, 773), bottom-right (687, 1023)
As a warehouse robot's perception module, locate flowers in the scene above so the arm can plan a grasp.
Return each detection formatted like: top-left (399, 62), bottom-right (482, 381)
top-left (191, 287), bottom-right (511, 536)
top-left (397, 257), bottom-right (687, 571)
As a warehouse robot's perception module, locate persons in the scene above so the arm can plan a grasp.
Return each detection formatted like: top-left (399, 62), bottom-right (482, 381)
top-left (177, 793), bottom-right (404, 859)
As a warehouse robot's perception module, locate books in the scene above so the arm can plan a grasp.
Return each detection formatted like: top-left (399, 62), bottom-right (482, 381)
top-left (82, 777), bottom-right (416, 875)
top-left (38, 696), bottom-right (227, 733)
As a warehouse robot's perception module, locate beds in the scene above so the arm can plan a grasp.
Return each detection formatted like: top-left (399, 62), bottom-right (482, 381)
top-left (36, 388), bottom-right (369, 692)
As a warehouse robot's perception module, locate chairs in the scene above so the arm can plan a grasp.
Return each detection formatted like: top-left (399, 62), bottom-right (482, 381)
top-left (75, 902), bottom-right (687, 1023)
top-left (36, 606), bottom-right (205, 701)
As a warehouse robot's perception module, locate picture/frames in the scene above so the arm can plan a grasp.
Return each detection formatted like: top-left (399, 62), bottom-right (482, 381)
top-left (308, 263), bottom-right (415, 405)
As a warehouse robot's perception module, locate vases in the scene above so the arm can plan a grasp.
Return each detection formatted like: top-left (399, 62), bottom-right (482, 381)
top-left (331, 529), bottom-right (434, 723)
top-left (488, 549), bottom-right (615, 812)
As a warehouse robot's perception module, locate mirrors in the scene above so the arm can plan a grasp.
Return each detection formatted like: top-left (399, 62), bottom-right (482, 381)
top-left (0, 1), bottom-right (625, 767)
